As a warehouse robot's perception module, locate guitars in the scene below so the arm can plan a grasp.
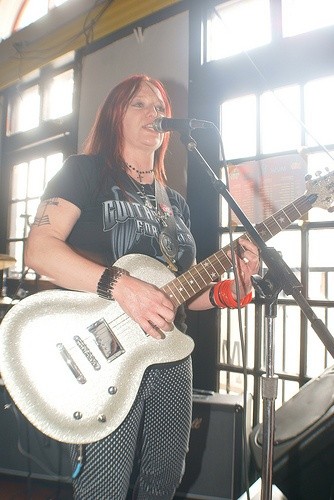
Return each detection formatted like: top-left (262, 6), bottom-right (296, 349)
top-left (1, 166), bottom-right (334, 446)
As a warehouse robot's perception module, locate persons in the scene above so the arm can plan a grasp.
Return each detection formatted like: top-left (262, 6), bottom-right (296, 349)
top-left (21, 75), bottom-right (266, 500)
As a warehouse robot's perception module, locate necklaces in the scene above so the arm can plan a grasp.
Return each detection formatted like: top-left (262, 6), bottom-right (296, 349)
top-left (124, 162), bottom-right (155, 182)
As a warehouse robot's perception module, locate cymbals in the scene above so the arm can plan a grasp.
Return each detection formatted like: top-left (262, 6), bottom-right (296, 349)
top-left (0, 254), bottom-right (17, 271)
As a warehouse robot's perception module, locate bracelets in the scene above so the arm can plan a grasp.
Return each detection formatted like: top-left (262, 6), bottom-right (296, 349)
top-left (97, 265), bottom-right (129, 302)
top-left (208, 281), bottom-right (253, 309)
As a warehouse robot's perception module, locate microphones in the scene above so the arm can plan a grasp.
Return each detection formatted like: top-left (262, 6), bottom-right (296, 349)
top-left (152, 115), bottom-right (215, 133)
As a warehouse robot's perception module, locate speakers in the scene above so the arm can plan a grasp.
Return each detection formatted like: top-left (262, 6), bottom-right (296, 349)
top-left (248, 362), bottom-right (333, 499)
top-left (173, 394), bottom-right (254, 500)
